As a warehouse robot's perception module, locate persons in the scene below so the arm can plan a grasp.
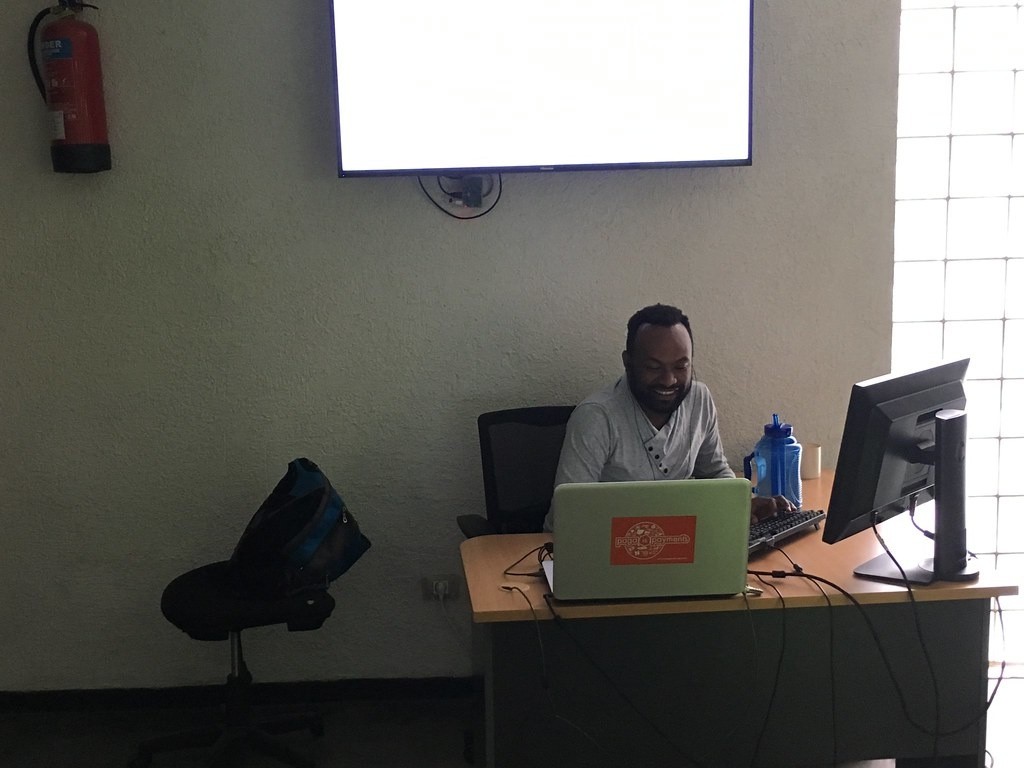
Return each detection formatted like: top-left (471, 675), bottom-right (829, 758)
top-left (544, 305), bottom-right (797, 533)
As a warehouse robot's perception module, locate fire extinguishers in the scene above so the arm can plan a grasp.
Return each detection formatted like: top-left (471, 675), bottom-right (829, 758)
top-left (27, 0), bottom-right (113, 174)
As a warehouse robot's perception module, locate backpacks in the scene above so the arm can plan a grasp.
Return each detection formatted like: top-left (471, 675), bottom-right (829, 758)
top-left (230, 458), bottom-right (371, 600)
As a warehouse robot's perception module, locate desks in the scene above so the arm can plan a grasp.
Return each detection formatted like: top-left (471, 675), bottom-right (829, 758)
top-left (460, 470), bottom-right (1018, 768)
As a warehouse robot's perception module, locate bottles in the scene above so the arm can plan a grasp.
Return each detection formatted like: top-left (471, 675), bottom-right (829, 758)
top-left (743, 413), bottom-right (803, 510)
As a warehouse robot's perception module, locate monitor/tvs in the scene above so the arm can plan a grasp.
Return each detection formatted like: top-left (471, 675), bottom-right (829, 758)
top-left (822, 354), bottom-right (980, 587)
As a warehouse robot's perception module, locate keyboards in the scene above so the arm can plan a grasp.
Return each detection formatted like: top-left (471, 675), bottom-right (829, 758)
top-left (748, 510), bottom-right (825, 556)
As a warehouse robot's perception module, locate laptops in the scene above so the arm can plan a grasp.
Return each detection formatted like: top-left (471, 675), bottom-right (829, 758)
top-left (542, 478), bottom-right (751, 602)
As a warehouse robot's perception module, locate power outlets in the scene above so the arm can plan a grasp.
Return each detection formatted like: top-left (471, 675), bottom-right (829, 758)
top-left (422, 574), bottom-right (461, 601)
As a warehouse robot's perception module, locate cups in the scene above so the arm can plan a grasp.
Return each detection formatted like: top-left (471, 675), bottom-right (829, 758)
top-left (800, 441), bottom-right (821, 479)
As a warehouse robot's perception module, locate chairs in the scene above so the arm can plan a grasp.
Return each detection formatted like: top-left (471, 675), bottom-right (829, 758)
top-left (127, 457), bottom-right (373, 768)
top-left (456, 405), bottom-right (574, 539)
